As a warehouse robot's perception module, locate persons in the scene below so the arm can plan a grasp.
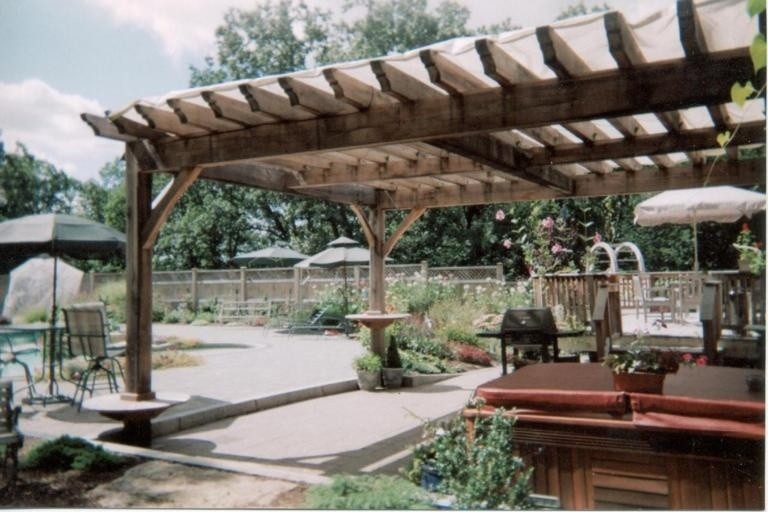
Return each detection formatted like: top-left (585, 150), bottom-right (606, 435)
top-left (634, 186), bottom-right (766, 274)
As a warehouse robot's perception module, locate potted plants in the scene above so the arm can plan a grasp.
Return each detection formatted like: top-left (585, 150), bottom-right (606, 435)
top-left (350, 333), bottom-right (405, 391)
top-left (600, 328), bottom-right (680, 394)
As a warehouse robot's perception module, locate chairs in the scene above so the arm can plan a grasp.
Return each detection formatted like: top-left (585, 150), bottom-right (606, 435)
top-left (60, 300), bottom-right (128, 413)
top-left (0, 331), bottom-right (43, 401)
top-left (631, 273), bottom-right (674, 322)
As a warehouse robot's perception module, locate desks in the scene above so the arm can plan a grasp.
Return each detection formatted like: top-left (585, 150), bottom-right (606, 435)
top-left (0, 320), bottom-right (72, 406)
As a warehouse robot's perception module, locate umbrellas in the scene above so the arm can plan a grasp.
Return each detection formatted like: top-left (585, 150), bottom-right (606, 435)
top-left (0, 205), bottom-right (128, 393)
top-left (293, 232), bottom-right (394, 312)
top-left (231, 243), bottom-right (308, 267)
